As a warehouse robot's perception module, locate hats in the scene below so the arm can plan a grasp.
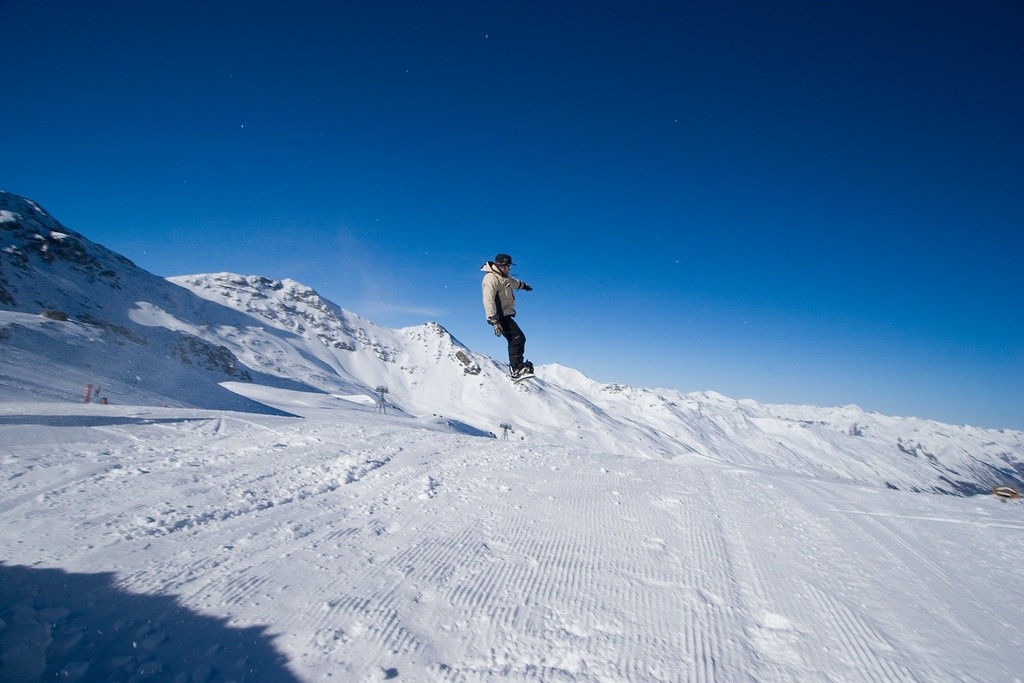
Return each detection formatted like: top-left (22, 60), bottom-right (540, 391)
top-left (495, 254), bottom-right (516, 266)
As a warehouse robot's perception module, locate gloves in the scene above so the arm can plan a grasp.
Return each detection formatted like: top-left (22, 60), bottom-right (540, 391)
top-left (522, 282), bottom-right (532, 291)
top-left (489, 314), bottom-right (503, 337)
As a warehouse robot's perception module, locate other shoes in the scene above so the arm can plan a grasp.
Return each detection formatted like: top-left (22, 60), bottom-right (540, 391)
top-left (507, 360), bottom-right (534, 378)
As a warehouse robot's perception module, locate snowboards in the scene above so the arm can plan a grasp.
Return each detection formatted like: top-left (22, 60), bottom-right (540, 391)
top-left (513, 372), bottom-right (536, 385)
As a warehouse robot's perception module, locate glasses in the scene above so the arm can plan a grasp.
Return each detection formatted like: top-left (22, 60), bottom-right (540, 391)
top-left (507, 264), bottom-right (510, 268)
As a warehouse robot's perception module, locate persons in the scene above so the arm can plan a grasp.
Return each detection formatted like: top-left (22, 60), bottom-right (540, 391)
top-left (481, 253), bottom-right (535, 377)
